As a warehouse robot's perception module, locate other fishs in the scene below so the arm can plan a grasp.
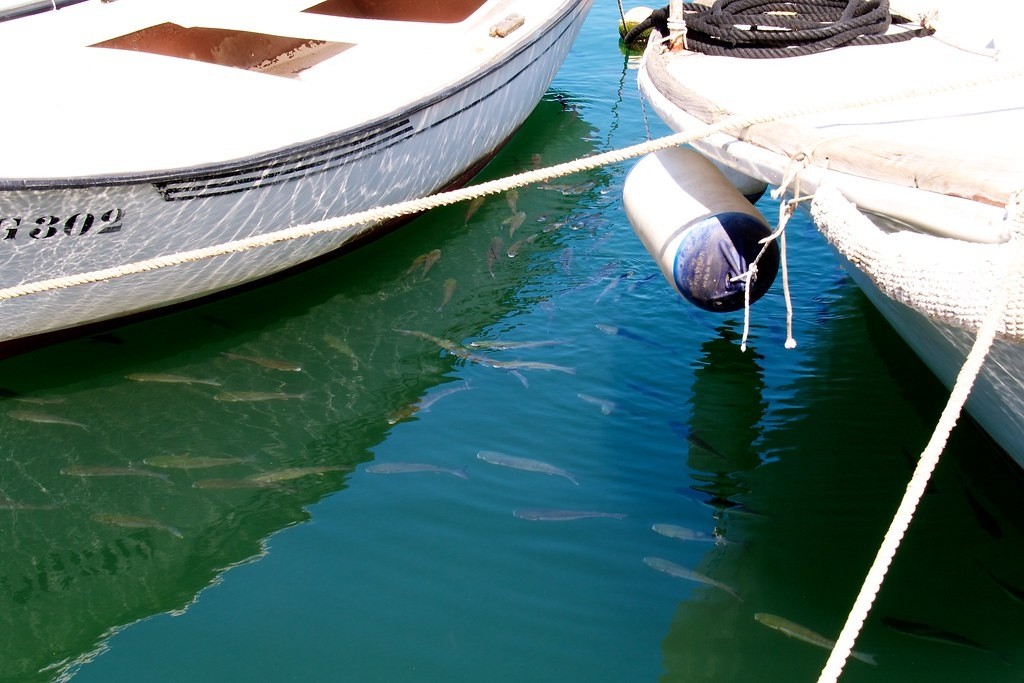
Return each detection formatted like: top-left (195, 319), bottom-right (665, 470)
top-left (0, 152), bottom-right (1023, 667)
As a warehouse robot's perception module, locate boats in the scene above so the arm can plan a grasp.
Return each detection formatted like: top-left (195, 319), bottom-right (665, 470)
top-left (0, 0), bottom-right (590, 337)
top-left (614, 0), bottom-right (1022, 456)
